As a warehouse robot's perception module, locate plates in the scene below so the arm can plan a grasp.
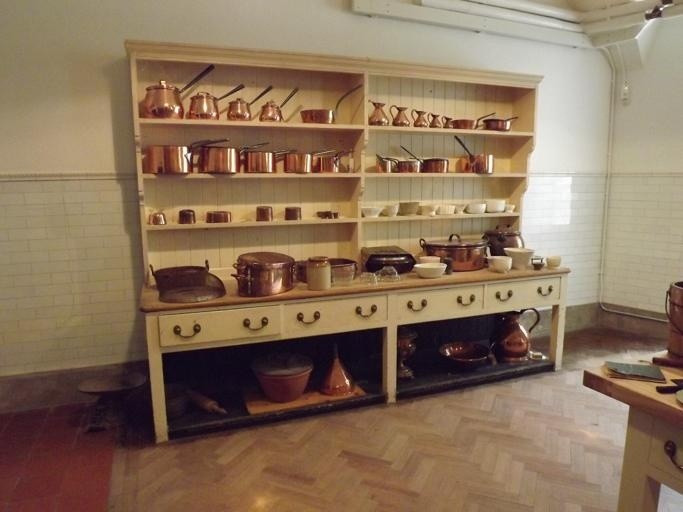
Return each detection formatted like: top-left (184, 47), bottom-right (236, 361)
top-left (676, 390), bottom-right (683, 404)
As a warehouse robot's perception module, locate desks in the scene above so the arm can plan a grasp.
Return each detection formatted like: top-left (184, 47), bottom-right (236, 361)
top-left (579, 353), bottom-right (681, 510)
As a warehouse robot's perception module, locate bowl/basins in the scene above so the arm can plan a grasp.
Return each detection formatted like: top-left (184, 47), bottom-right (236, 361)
top-left (177, 209), bottom-right (196, 225)
top-left (488, 255), bottom-right (511, 273)
top-left (415, 262), bottom-right (446, 277)
top-left (206, 211), bottom-right (232, 223)
top-left (418, 255), bottom-right (441, 264)
top-left (360, 198), bottom-right (515, 219)
top-left (439, 340), bottom-right (490, 368)
top-left (504, 246), bottom-right (535, 270)
top-left (546, 256), bottom-right (561, 268)
top-left (255, 206), bottom-right (273, 222)
top-left (148, 213), bottom-right (165, 224)
top-left (254, 362), bottom-right (315, 401)
top-left (284, 207), bottom-right (301, 220)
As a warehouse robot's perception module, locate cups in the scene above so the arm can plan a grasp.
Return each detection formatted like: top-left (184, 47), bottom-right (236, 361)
top-left (317, 210), bottom-right (339, 218)
top-left (359, 272), bottom-right (377, 286)
top-left (377, 266), bottom-right (399, 282)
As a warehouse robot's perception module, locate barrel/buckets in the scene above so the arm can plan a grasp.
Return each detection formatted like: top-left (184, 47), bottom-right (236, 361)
top-left (664, 280), bottom-right (683, 359)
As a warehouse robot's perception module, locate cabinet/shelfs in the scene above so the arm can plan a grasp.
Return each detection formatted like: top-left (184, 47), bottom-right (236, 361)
top-left (122, 39), bottom-right (570, 446)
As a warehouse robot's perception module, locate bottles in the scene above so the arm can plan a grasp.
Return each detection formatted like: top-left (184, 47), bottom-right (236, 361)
top-left (319, 341), bottom-right (354, 397)
top-left (306, 257), bottom-right (332, 291)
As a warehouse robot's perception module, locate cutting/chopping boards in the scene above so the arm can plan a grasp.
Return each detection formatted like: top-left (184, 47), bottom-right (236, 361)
top-left (651, 350), bottom-right (683, 370)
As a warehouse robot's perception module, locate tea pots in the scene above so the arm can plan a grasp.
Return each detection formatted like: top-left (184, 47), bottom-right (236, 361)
top-left (491, 308), bottom-right (540, 364)
top-left (367, 100), bottom-right (452, 128)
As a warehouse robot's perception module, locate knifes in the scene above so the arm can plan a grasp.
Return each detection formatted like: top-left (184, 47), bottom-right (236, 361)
top-left (656, 377), bottom-right (682, 395)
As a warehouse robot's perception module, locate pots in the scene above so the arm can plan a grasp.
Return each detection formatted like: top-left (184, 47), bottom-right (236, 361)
top-left (399, 144), bottom-right (446, 173)
top-left (149, 259), bottom-right (208, 292)
top-left (300, 84), bottom-right (363, 123)
top-left (382, 157), bottom-right (421, 173)
top-left (483, 223), bottom-right (524, 256)
top-left (229, 252), bottom-right (296, 295)
top-left (143, 139), bottom-right (356, 175)
top-left (143, 64), bottom-right (299, 122)
top-left (453, 113), bottom-right (496, 129)
top-left (482, 116), bottom-right (517, 130)
top-left (453, 134), bottom-right (492, 173)
top-left (298, 256), bottom-right (356, 284)
top-left (376, 153), bottom-right (391, 173)
top-left (417, 233), bottom-right (491, 270)
